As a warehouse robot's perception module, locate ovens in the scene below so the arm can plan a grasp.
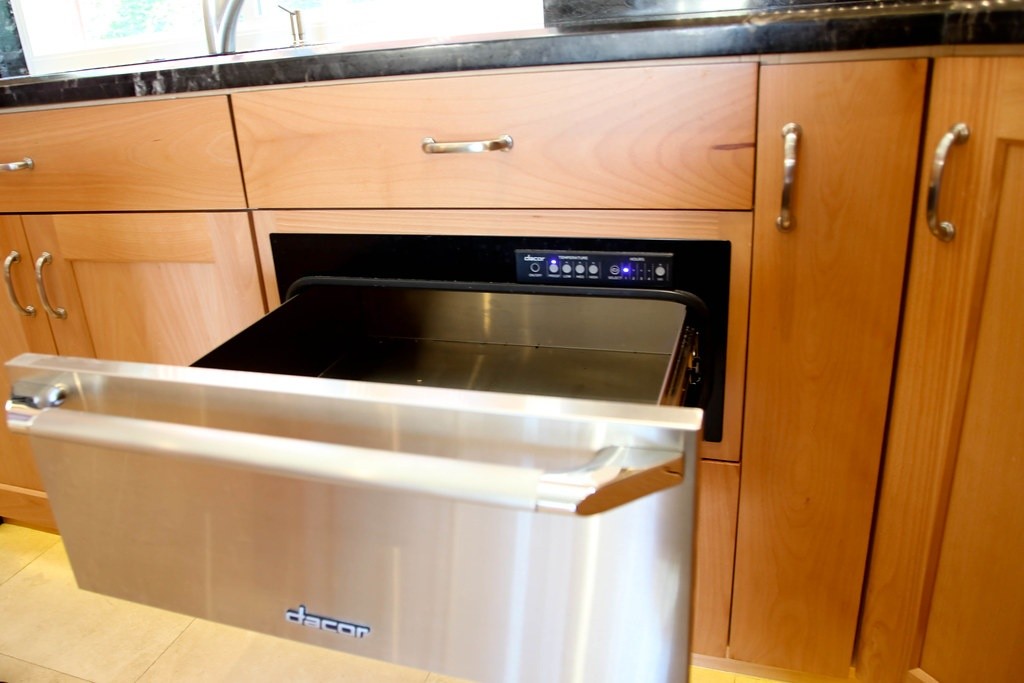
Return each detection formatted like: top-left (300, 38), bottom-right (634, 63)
top-left (2, 227), bottom-right (715, 683)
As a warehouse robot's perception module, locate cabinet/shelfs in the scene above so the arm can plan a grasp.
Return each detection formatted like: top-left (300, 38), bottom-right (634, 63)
top-left (0, 47), bottom-right (1024, 683)
